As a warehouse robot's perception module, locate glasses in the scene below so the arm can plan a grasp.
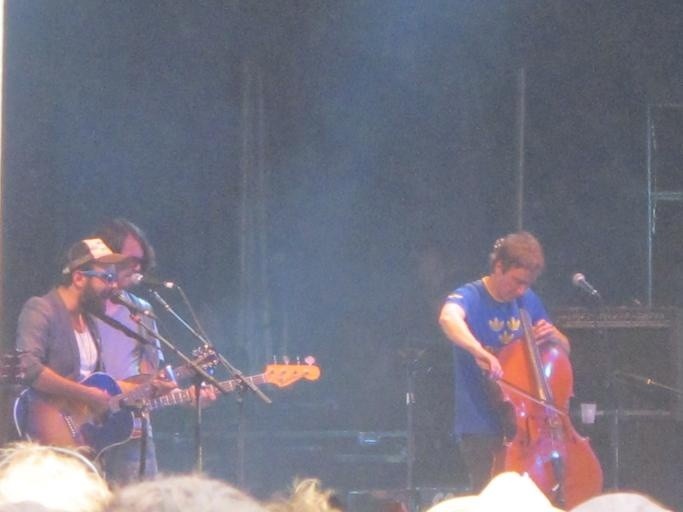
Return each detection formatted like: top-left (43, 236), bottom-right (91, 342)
top-left (80, 271), bottom-right (114, 283)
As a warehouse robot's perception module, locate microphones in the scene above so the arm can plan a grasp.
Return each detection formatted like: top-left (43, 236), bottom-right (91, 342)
top-left (572, 272), bottom-right (599, 297)
top-left (615, 368), bottom-right (681, 406)
top-left (110, 290), bottom-right (160, 321)
top-left (128, 273), bottom-right (176, 289)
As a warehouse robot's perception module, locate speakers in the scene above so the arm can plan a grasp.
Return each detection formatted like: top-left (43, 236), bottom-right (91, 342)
top-left (612, 95), bottom-right (682, 309)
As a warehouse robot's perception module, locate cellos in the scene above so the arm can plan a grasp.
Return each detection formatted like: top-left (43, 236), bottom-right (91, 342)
top-left (487, 238), bottom-right (603, 507)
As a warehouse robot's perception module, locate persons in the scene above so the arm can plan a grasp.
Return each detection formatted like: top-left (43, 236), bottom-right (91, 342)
top-left (11, 235), bottom-right (179, 488)
top-left (437, 230), bottom-right (571, 498)
top-left (82, 215), bottom-right (220, 483)
top-left (0, 442), bottom-right (681, 512)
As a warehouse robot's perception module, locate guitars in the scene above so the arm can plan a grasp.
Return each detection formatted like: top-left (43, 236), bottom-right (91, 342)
top-left (118, 356), bottom-right (320, 441)
top-left (6, 348), bottom-right (219, 463)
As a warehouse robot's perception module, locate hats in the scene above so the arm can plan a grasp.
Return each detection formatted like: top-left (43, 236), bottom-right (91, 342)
top-left (70, 238), bottom-right (128, 270)
